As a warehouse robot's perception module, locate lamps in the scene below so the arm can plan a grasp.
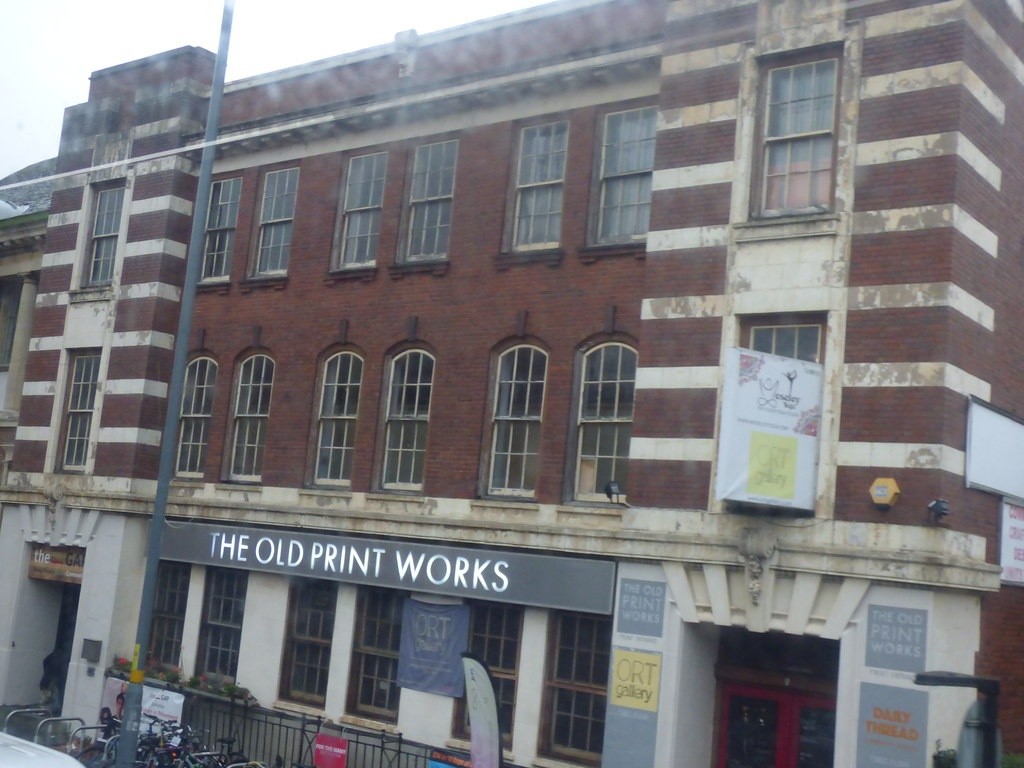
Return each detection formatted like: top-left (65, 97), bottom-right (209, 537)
top-left (928, 496), bottom-right (949, 524)
top-left (605, 480), bottom-right (620, 505)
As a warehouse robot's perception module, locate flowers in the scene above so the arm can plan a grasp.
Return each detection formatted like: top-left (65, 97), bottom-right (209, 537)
top-left (112, 654), bottom-right (250, 701)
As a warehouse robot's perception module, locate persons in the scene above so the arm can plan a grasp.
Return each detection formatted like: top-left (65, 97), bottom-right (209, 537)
top-left (101, 683), bottom-right (129, 740)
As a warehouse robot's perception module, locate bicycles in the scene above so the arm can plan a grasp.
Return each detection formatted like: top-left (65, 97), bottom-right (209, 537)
top-left (76, 714), bottom-right (267, 768)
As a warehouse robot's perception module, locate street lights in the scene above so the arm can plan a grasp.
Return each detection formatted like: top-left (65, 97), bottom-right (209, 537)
top-left (911, 669), bottom-right (997, 767)
